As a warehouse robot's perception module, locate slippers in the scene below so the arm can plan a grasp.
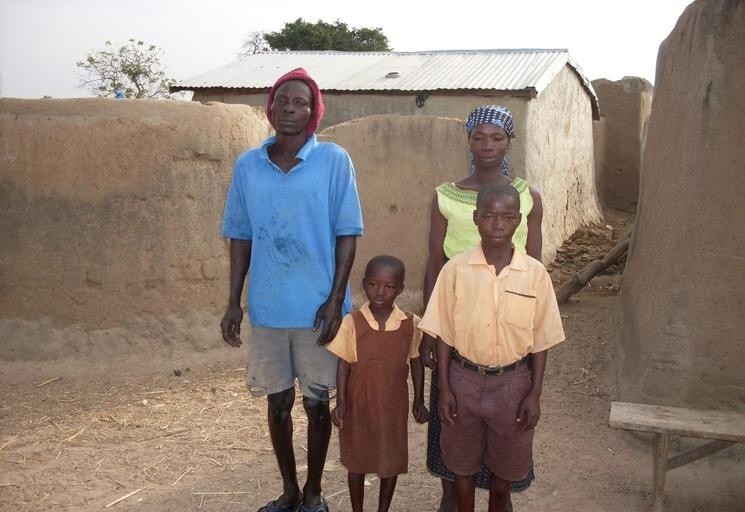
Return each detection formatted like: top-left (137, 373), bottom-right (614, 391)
top-left (258, 494), bottom-right (328, 512)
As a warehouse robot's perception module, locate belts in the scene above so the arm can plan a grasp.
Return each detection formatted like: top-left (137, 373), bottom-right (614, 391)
top-left (453, 349), bottom-right (528, 376)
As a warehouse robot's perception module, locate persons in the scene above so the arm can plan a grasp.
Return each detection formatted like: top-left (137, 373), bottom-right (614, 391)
top-left (221, 68), bottom-right (364, 512)
top-left (419, 104), bottom-right (543, 512)
top-left (326, 256), bottom-right (430, 512)
top-left (415, 182), bottom-right (565, 512)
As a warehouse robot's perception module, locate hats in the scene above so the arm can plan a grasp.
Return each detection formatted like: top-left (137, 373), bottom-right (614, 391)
top-left (264, 67), bottom-right (324, 138)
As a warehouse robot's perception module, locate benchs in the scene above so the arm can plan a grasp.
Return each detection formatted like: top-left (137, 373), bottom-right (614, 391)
top-left (607, 400), bottom-right (744, 512)
